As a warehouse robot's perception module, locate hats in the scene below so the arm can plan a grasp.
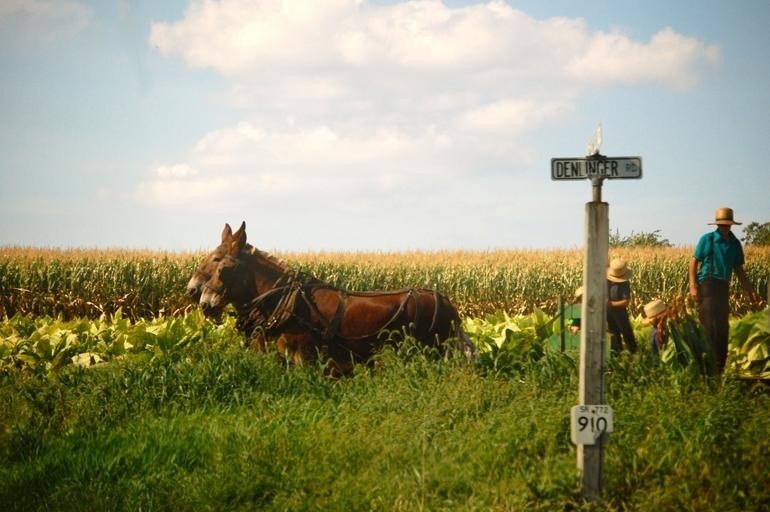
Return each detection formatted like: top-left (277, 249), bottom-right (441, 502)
top-left (643, 300), bottom-right (671, 323)
top-left (708, 208), bottom-right (742, 225)
top-left (607, 260), bottom-right (633, 282)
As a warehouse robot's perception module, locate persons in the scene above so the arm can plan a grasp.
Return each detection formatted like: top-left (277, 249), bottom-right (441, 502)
top-left (689, 208), bottom-right (761, 372)
top-left (644, 299), bottom-right (679, 367)
top-left (606, 260), bottom-right (638, 357)
top-left (568, 287), bottom-right (583, 335)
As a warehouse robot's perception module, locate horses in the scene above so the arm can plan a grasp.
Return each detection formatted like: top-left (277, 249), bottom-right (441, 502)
top-left (200, 230), bottom-right (462, 378)
top-left (186, 221), bottom-right (352, 376)
